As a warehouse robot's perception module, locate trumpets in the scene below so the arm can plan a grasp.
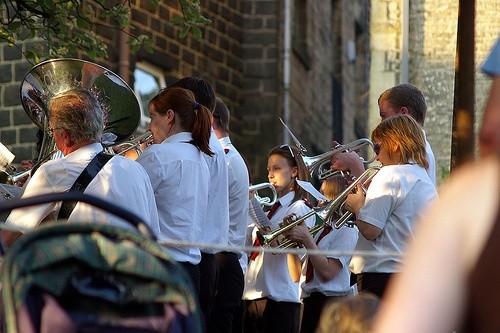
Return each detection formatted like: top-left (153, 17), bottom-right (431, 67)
top-left (256, 211), bottom-right (326, 256)
top-left (314, 165), bottom-right (383, 231)
top-left (241, 180), bottom-right (278, 232)
top-left (297, 138), bottom-right (378, 179)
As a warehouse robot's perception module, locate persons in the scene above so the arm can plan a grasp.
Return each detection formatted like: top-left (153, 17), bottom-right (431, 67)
top-left (174, 76), bottom-right (230, 333)
top-left (0, 88), bottom-right (161, 254)
top-left (210, 95), bottom-right (250, 333)
top-left (316, 32), bottom-right (500, 333)
top-left (279, 175), bottom-right (359, 333)
top-left (331, 84), bottom-right (437, 189)
top-left (346, 115), bottom-right (439, 301)
top-left (243, 144), bottom-right (316, 333)
top-left (134, 87), bottom-right (216, 333)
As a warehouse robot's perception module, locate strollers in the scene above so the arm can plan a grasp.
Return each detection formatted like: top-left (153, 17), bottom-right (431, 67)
top-left (0, 192), bottom-right (205, 333)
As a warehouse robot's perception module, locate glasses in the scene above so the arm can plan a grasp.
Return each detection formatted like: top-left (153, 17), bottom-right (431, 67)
top-left (45, 126), bottom-right (70, 138)
top-left (280, 144), bottom-right (294, 157)
top-left (374, 143), bottom-right (384, 155)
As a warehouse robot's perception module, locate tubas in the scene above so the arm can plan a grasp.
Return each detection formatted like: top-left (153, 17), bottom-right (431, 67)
top-left (0, 58), bottom-right (153, 186)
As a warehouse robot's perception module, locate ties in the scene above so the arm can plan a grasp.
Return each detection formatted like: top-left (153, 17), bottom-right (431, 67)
top-left (249, 201), bottom-right (281, 260)
top-left (306, 226), bottom-right (332, 283)
